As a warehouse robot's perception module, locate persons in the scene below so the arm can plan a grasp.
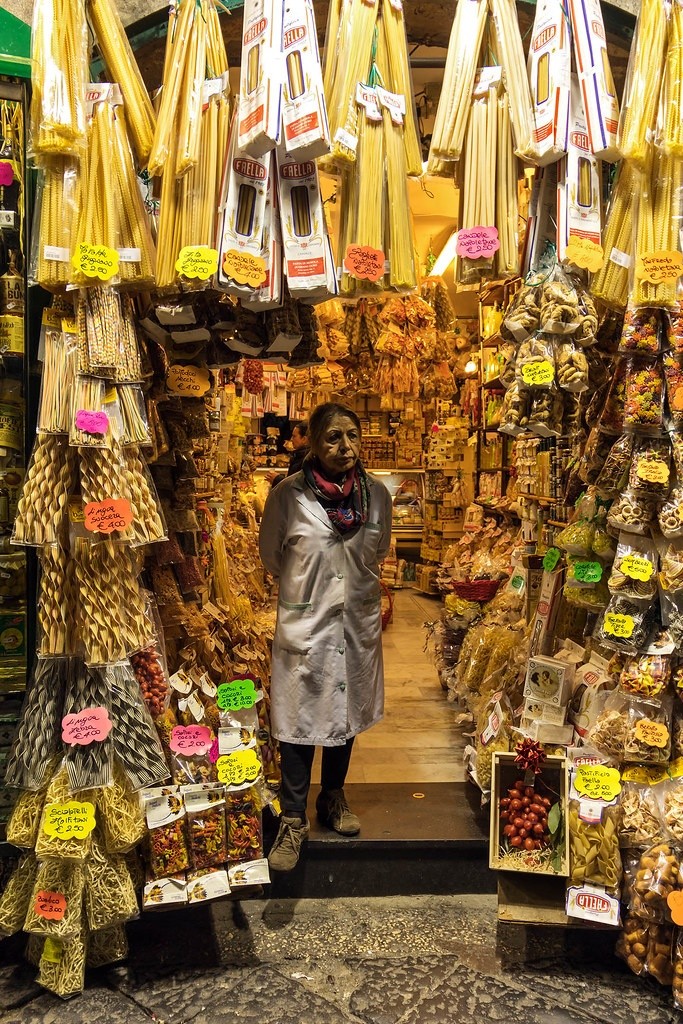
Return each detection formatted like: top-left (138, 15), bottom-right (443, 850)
top-left (263, 420), bottom-right (312, 488)
top-left (259, 403), bottom-right (394, 873)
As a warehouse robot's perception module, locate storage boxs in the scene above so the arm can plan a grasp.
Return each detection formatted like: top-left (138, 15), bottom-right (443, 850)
top-left (214, 0), bottom-right (340, 314)
top-left (491, 751), bottom-right (570, 878)
top-left (0, 552), bottom-right (28, 693)
top-left (527, 0), bottom-right (621, 274)
top-left (518, 654), bottom-right (575, 745)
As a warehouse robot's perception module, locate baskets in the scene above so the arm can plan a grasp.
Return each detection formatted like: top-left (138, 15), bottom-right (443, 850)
top-left (379, 579), bottom-right (394, 631)
top-left (452, 577), bottom-right (502, 602)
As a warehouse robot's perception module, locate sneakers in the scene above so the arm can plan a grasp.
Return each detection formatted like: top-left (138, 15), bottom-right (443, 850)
top-left (267, 816), bottom-right (310, 873)
top-left (315, 788), bottom-right (361, 837)
